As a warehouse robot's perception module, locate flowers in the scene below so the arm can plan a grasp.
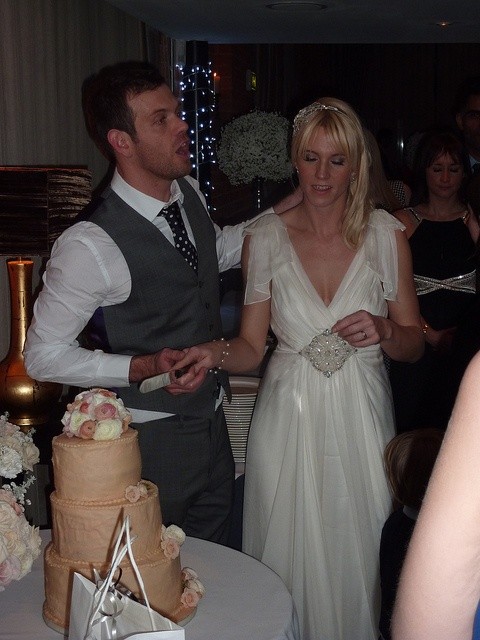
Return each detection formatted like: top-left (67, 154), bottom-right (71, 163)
top-left (0, 411), bottom-right (40, 594)
top-left (217, 110), bottom-right (295, 183)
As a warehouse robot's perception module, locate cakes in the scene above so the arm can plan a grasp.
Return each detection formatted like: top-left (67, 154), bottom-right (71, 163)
top-left (41, 388), bottom-right (205, 635)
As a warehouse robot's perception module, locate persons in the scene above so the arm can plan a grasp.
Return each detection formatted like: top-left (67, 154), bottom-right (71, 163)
top-left (392, 137), bottom-right (480, 430)
top-left (393, 344), bottom-right (480, 640)
top-left (379, 428), bottom-right (437, 640)
top-left (374, 130), bottom-right (413, 207)
top-left (174, 98), bottom-right (425, 640)
top-left (22, 61), bottom-right (304, 551)
top-left (457, 96), bottom-right (480, 210)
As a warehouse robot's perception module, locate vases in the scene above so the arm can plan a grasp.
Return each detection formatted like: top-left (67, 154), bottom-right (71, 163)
top-left (0, 258), bottom-right (63, 426)
top-left (253, 176), bottom-right (264, 217)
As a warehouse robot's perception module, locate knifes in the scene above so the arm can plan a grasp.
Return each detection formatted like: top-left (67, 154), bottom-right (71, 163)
top-left (138, 361), bottom-right (194, 394)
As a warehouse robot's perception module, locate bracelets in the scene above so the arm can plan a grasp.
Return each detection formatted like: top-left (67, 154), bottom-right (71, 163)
top-left (207, 336), bottom-right (232, 375)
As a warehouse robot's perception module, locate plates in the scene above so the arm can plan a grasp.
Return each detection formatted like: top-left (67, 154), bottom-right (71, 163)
top-left (222, 376), bottom-right (262, 464)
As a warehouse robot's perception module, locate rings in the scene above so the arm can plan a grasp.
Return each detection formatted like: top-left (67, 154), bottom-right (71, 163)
top-left (360, 331), bottom-right (369, 340)
